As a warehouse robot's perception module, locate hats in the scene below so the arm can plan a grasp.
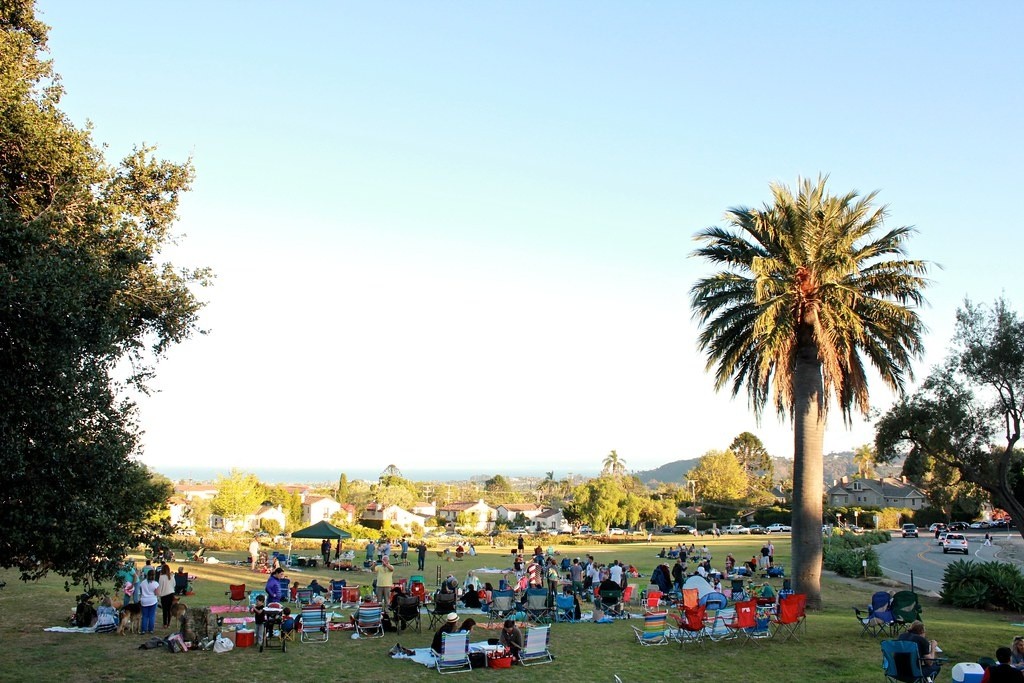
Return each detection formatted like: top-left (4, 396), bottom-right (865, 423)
top-left (446, 612), bottom-right (460, 622)
top-left (365, 595), bottom-right (373, 600)
top-left (390, 587), bottom-right (401, 592)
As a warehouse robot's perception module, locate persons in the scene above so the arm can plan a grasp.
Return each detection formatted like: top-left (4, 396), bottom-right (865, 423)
top-left (752, 555), bottom-right (756, 565)
top-left (253, 567), bottom-right (335, 648)
top-left (662, 558), bottom-right (687, 591)
top-left (983, 531), bottom-right (991, 546)
top-left (321, 538), bottom-right (343, 564)
top-left (436, 571), bottom-right (493, 614)
top-left (431, 612), bottom-right (476, 657)
top-left (352, 536), bottom-right (410, 632)
top-left (500, 620), bottom-right (522, 665)
top-left (415, 541), bottom-right (426, 570)
top-left (74, 559), bottom-right (186, 634)
top-left (660, 542), bottom-right (711, 562)
top-left (899, 620), bottom-right (941, 681)
top-left (518, 534), bottom-right (524, 553)
top-left (647, 533), bottom-right (652, 543)
top-left (761, 540), bottom-right (774, 566)
top-left (981, 635), bottom-right (1024, 683)
top-left (726, 553), bottom-right (735, 567)
top-left (696, 559), bottom-right (721, 592)
top-left (742, 563), bottom-right (752, 576)
top-left (513, 545), bottom-right (638, 621)
top-left (249, 537), bottom-right (260, 571)
top-left (447, 540), bottom-right (476, 562)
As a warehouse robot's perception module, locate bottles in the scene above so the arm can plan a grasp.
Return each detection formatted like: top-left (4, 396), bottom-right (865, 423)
top-left (243, 621), bottom-right (246, 630)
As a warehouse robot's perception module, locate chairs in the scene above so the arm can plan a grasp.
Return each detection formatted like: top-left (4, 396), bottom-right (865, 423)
top-left (178, 572), bottom-right (947, 683)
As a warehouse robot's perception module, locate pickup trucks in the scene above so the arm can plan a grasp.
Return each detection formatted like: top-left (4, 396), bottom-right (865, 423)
top-left (766, 523), bottom-right (792, 532)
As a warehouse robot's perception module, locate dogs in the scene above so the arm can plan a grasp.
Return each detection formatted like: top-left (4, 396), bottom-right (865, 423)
top-left (116, 601), bottom-right (141, 636)
top-left (171, 597), bottom-right (187, 628)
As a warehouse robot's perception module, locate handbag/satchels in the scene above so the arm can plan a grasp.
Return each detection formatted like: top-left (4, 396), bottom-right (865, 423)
top-left (465, 653), bottom-right (488, 667)
top-left (164, 633), bottom-right (183, 653)
top-left (213, 638), bottom-right (234, 653)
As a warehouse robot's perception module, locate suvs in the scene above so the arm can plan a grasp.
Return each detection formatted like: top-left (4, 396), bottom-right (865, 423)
top-left (672, 525), bottom-right (694, 535)
top-left (900, 523), bottom-right (919, 538)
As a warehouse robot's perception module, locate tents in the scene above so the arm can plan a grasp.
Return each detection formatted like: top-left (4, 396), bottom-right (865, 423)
top-left (288, 520), bottom-right (352, 570)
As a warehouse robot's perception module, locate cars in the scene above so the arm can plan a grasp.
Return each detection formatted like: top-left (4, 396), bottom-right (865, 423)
top-left (942, 532), bottom-right (969, 556)
top-left (970, 521), bottom-right (991, 530)
top-left (609, 527), bottom-right (646, 536)
top-left (703, 524), bottom-right (750, 535)
top-left (660, 525), bottom-right (673, 533)
top-left (937, 532), bottom-right (948, 545)
top-left (487, 526), bottom-right (595, 536)
top-left (989, 517), bottom-right (1017, 528)
top-left (749, 523), bottom-right (766, 534)
top-left (929, 521), bottom-right (970, 540)
